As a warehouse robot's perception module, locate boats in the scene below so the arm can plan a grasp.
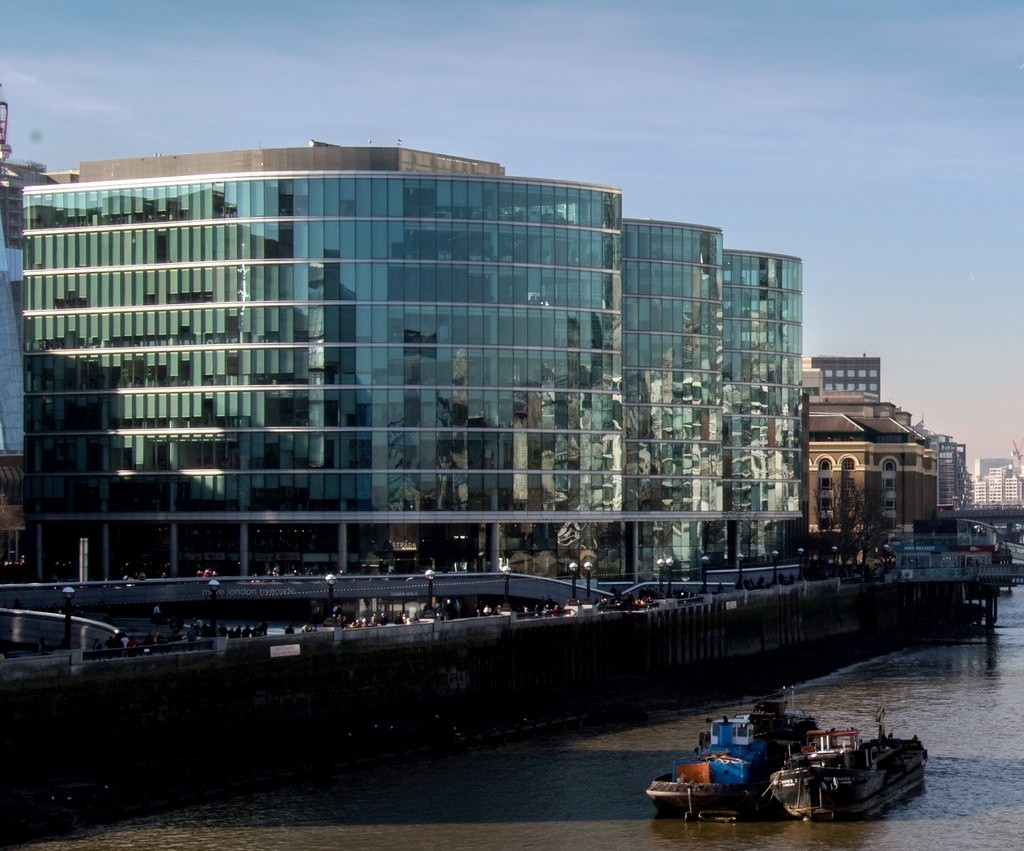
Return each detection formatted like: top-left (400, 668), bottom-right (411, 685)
top-left (643, 696), bottom-right (818, 821)
top-left (772, 702), bottom-right (929, 824)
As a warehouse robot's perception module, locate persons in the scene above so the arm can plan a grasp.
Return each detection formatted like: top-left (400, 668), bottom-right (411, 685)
top-left (698, 556), bottom-right (896, 594)
top-left (0, 561), bottom-right (696, 660)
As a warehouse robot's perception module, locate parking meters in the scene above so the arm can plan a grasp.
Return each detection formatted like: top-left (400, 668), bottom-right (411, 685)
top-left (501, 565), bottom-right (510, 610)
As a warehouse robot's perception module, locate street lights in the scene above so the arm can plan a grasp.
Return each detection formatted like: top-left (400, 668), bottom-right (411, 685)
top-left (797, 548), bottom-right (805, 581)
top-left (772, 551), bottom-right (779, 586)
top-left (884, 545), bottom-right (889, 573)
top-left (583, 562), bottom-right (593, 598)
top-left (326, 574), bottom-right (337, 626)
top-left (830, 546), bottom-right (837, 578)
top-left (665, 559), bottom-right (674, 595)
top-left (702, 556), bottom-right (708, 594)
top-left (425, 570), bottom-right (436, 610)
top-left (569, 562), bottom-right (578, 600)
top-left (62, 587), bottom-right (76, 650)
top-left (657, 559), bottom-right (665, 594)
top-left (737, 554), bottom-right (745, 590)
top-left (209, 580), bottom-right (221, 636)
